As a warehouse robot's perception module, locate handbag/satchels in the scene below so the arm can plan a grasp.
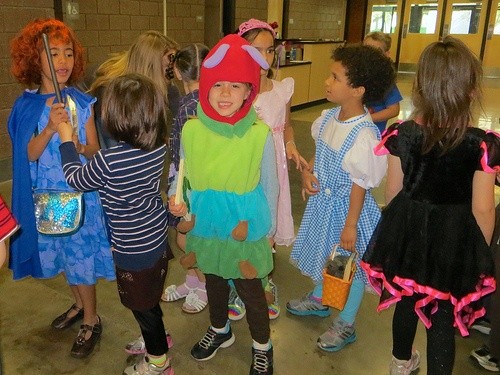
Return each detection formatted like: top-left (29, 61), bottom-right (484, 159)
top-left (32, 186), bottom-right (87, 238)
top-left (321, 243), bottom-right (360, 313)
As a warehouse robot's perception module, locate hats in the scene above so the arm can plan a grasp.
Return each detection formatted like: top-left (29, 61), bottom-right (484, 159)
top-left (198, 32), bottom-right (269, 126)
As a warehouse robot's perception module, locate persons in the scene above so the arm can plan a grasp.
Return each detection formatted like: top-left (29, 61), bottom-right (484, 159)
top-left (357, 32), bottom-right (404, 136)
top-left (167, 34), bottom-right (280, 375)
top-left (55, 74), bottom-right (174, 375)
top-left (0, 197), bottom-right (20, 270)
top-left (84, 19), bottom-right (310, 320)
top-left (360, 37), bottom-right (500, 375)
top-left (284, 44), bottom-right (395, 352)
top-left (7, 16), bottom-right (117, 358)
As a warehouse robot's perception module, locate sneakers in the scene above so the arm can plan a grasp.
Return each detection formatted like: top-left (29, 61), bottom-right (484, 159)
top-left (121, 332), bottom-right (175, 356)
top-left (245, 339), bottom-right (275, 375)
top-left (314, 316), bottom-right (358, 352)
top-left (466, 316), bottom-right (500, 372)
top-left (389, 348), bottom-right (421, 375)
top-left (284, 290), bottom-right (330, 318)
top-left (123, 351), bottom-right (175, 375)
top-left (189, 323), bottom-right (236, 361)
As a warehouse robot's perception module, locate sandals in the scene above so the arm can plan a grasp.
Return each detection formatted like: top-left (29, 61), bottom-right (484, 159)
top-left (160, 281), bottom-right (194, 302)
top-left (181, 287), bottom-right (209, 314)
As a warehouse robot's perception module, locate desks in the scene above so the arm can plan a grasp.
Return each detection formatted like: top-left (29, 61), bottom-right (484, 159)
top-left (294, 39), bottom-right (348, 107)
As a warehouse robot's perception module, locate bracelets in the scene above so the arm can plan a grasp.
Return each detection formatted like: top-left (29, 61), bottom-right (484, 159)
top-left (287, 141), bottom-right (296, 147)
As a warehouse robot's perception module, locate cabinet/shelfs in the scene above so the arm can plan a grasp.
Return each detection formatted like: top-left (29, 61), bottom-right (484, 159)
top-left (276, 61), bottom-right (312, 112)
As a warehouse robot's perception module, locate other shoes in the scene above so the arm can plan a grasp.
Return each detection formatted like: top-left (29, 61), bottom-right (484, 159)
top-left (224, 287), bottom-right (246, 321)
top-left (70, 315), bottom-right (102, 358)
top-left (263, 276), bottom-right (282, 320)
top-left (50, 303), bottom-right (83, 331)
